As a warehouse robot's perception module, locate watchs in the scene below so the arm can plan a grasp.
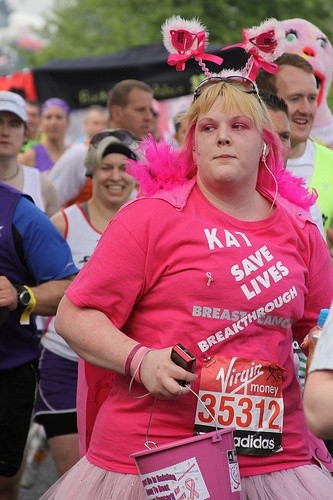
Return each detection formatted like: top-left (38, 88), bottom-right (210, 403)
top-left (14, 284), bottom-right (31, 314)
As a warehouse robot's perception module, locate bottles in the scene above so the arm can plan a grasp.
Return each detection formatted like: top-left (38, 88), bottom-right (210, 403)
top-left (304, 309), bottom-right (330, 396)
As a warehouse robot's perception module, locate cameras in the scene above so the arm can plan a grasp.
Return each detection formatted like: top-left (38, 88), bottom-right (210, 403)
top-left (171, 343), bottom-right (196, 387)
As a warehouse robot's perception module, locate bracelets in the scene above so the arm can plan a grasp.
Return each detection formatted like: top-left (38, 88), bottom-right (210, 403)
top-left (123, 343), bottom-right (152, 385)
top-left (20, 286), bottom-right (36, 325)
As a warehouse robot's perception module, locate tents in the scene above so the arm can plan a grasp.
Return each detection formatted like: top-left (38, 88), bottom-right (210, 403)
top-left (0, 36), bottom-right (252, 112)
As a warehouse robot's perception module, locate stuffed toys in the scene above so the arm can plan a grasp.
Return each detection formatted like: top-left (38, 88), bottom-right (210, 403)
top-left (244, 18), bottom-right (333, 149)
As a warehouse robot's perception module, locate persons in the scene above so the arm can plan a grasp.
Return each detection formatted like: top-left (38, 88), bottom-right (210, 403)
top-left (258, 88), bottom-right (326, 241)
top-left (31, 130), bottom-right (139, 479)
top-left (21, 79), bottom-right (153, 488)
top-left (251, 52), bottom-right (333, 394)
top-left (303, 299), bottom-right (333, 440)
top-left (0, 81), bottom-right (197, 221)
top-left (0, 181), bottom-right (79, 500)
top-left (55, 69), bottom-right (333, 500)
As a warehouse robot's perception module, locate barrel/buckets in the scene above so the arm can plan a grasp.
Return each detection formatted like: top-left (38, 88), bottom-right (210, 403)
top-left (130, 384), bottom-right (241, 500)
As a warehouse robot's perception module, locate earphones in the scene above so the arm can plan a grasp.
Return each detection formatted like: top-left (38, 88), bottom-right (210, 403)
top-left (263, 141), bottom-right (267, 155)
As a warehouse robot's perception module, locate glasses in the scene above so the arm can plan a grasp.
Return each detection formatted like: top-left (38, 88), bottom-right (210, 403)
top-left (89, 130), bottom-right (136, 148)
top-left (194, 76), bottom-right (262, 109)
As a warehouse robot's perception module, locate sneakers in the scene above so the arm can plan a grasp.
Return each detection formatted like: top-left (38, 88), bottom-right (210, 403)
top-left (20, 422), bottom-right (47, 489)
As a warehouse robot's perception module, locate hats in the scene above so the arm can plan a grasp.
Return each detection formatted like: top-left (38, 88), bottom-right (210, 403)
top-left (0, 91), bottom-right (28, 123)
